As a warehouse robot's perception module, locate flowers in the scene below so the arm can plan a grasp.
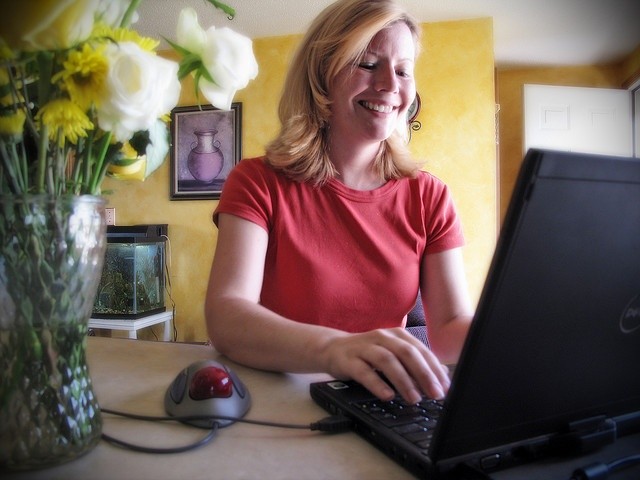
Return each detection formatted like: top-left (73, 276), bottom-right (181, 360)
top-left (1, 0), bottom-right (258, 470)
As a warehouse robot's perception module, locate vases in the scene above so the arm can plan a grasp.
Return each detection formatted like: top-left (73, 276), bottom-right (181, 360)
top-left (0, 192), bottom-right (108, 470)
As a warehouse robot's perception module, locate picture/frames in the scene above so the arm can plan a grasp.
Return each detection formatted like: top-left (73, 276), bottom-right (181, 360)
top-left (170, 101), bottom-right (243, 201)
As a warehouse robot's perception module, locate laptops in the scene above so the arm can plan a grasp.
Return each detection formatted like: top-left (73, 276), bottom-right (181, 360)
top-left (308, 147), bottom-right (640, 480)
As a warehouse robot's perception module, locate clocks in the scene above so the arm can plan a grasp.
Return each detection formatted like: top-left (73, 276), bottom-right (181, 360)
top-left (406, 93), bottom-right (423, 146)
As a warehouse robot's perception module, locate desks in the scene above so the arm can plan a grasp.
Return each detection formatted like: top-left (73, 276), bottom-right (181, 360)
top-left (87, 311), bottom-right (175, 341)
top-left (0, 337), bottom-right (427, 480)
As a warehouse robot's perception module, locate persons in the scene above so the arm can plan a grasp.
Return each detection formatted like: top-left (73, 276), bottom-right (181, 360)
top-left (204, 0), bottom-right (475, 405)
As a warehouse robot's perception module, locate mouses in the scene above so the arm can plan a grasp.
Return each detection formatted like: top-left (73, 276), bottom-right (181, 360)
top-left (164, 359), bottom-right (251, 429)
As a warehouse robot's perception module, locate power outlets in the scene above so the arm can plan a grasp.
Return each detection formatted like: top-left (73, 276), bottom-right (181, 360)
top-left (103, 208), bottom-right (117, 225)
